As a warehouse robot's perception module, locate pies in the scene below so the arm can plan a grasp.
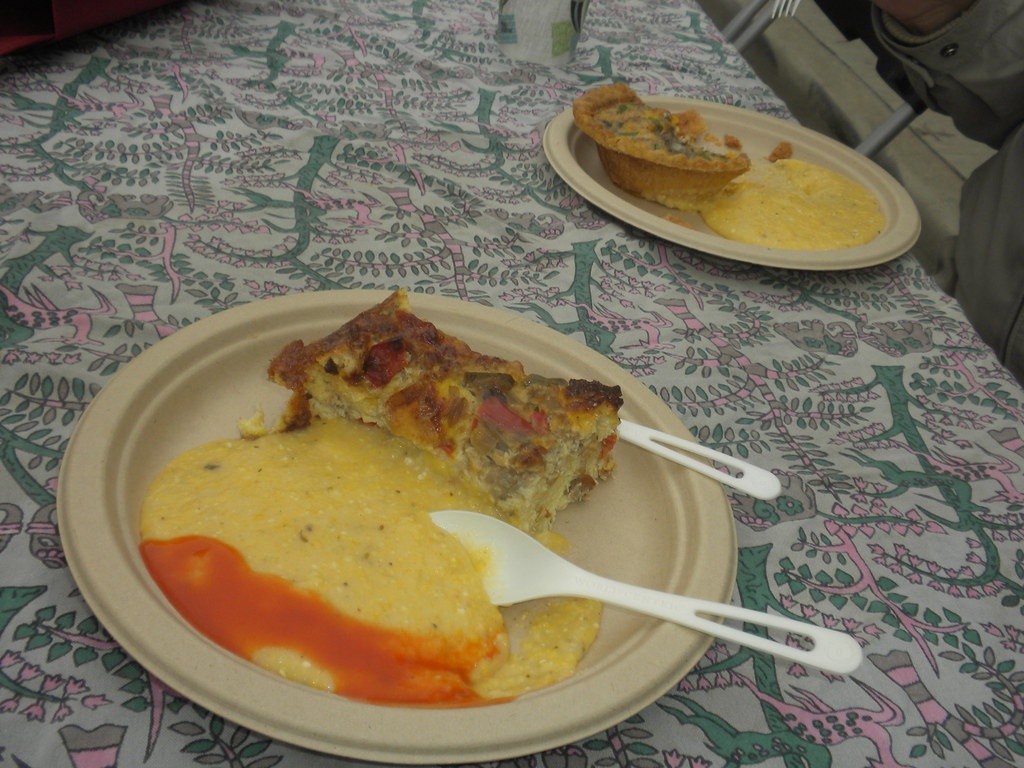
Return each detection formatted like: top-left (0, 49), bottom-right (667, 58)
top-left (571, 83), bottom-right (751, 209)
top-left (238, 290), bottom-right (621, 526)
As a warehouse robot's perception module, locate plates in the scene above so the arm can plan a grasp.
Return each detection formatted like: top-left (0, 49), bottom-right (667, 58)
top-left (543, 95), bottom-right (921, 271)
top-left (57, 290), bottom-right (739, 764)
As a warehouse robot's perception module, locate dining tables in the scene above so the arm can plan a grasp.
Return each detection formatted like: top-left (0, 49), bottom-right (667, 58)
top-left (0, 0), bottom-right (1024, 768)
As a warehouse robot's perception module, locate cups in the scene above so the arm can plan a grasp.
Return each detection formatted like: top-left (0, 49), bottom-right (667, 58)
top-left (497, 0), bottom-right (592, 67)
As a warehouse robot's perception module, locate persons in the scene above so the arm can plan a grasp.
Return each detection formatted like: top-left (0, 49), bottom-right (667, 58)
top-left (869, 0), bottom-right (1024, 390)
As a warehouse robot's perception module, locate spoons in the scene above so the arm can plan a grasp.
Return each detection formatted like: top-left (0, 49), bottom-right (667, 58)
top-left (427, 511), bottom-right (863, 674)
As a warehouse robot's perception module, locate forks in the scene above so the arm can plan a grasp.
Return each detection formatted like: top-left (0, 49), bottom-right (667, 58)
top-left (772, 0), bottom-right (800, 18)
